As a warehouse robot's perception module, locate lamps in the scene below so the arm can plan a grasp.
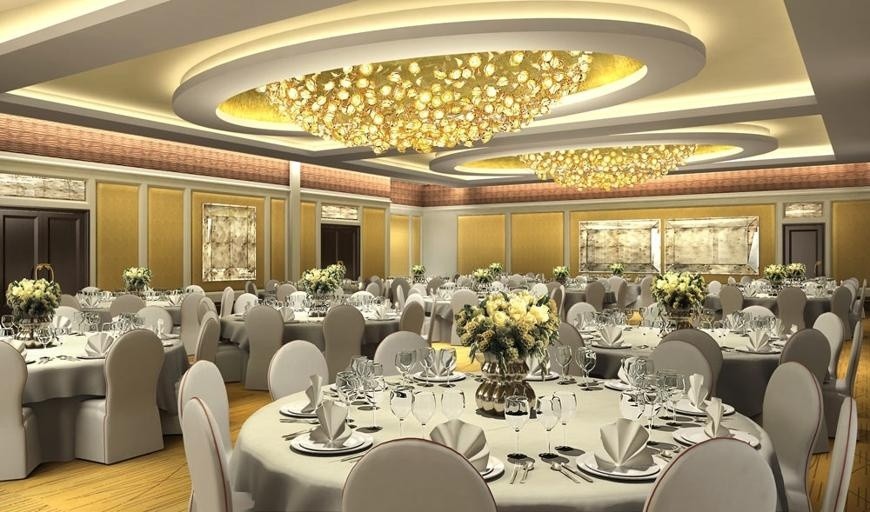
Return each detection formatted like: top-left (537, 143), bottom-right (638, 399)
top-left (255, 49), bottom-right (596, 158)
top-left (513, 144), bottom-right (699, 192)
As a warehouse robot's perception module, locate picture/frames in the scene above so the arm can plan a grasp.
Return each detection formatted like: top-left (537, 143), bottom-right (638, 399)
top-left (661, 215), bottom-right (760, 276)
top-left (200, 202), bottom-right (258, 282)
top-left (577, 218), bottom-right (664, 274)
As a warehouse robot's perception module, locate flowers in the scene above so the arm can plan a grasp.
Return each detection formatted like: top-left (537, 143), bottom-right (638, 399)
top-left (610, 262), bottom-right (624, 276)
top-left (300, 259), bottom-right (347, 294)
top-left (474, 262), bottom-right (503, 288)
top-left (648, 258), bottom-right (808, 326)
top-left (552, 264), bottom-right (570, 287)
top-left (412, 264), bottom-right (426, 285)
top-left (120, 265), bottom-right (153, 295)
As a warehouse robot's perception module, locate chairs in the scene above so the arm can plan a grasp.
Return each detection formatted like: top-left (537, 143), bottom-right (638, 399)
top-left (1, 271), bottom-right (870, 510)
